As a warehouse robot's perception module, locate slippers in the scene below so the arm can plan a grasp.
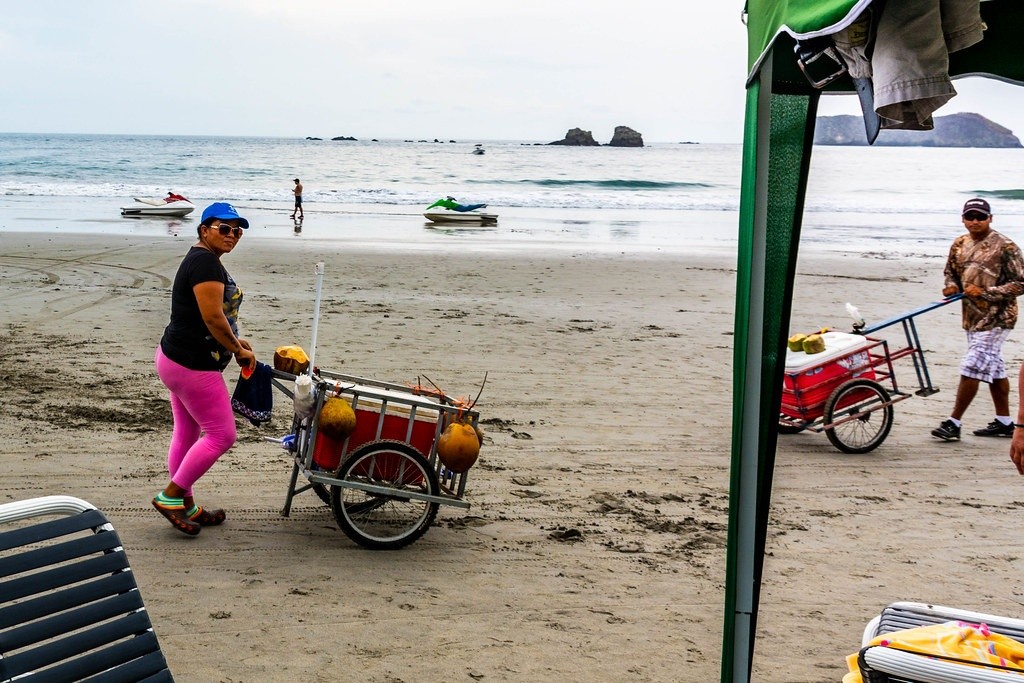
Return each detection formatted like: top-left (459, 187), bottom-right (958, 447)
top-left (151, 498), bottom-right (201, 535)
top-left (188, 505), bottom-right (226, 526)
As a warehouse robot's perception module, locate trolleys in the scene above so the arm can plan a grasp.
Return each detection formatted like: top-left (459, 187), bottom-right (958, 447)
top-left (239, 259), bottom-right (486, 552)
top-left (775, 287), bottom-right (972, 455)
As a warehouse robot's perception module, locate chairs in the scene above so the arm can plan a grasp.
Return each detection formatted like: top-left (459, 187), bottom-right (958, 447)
top-left (0, 494), bottom-right (174, 683)
top-left (858, 601), bottom-right (1024, 683)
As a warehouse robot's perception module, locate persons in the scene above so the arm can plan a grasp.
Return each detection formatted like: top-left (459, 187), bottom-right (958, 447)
top-left (1009, 361), bottom-right (1024, 476)
top-left (929, 199), bottom-right (1024, 442)
top-left (290, 179), bottom-right (304, 217)
top-left (151, 202), bottom-right (258, 535)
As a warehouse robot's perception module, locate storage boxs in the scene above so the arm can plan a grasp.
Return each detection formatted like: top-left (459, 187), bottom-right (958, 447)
top-left (781, 332), bottom-right (877, 419)
top-left (312, 379), bottom-right (439, 486)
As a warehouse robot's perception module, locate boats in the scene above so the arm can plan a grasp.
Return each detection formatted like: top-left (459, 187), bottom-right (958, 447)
top-left (472, 143), bottom-right (485, 155)
top-left (120, 196), bottom-right (195, 218)
top-left (424, 210), bottom-right (501, 223)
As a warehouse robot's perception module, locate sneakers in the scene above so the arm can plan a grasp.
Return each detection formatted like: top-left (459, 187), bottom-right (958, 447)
top-left (972, 418), bottom-right (1016, 437)
top-left (931, 420), bottom-right (961, 439)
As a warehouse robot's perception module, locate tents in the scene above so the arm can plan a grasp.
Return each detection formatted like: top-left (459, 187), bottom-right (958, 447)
top-left (717, 1), bottom-right (1024, 682)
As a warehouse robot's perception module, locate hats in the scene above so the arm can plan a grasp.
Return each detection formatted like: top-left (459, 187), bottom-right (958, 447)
top-left (293, 179), bottom-right (299, 183)
top-left (961, 198), bottom-right (990, 215)
top-left (201, 202), bottom-right (249, 229)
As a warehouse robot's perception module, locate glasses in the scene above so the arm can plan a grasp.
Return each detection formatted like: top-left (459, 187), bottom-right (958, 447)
top-left (210, 222), bottom-right (243, 239)
top-left (964, 212), bottom-right (988, 221)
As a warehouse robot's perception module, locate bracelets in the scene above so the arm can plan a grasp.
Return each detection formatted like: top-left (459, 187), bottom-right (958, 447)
top-left (1014, 424), bottom-right (1024, 428)
